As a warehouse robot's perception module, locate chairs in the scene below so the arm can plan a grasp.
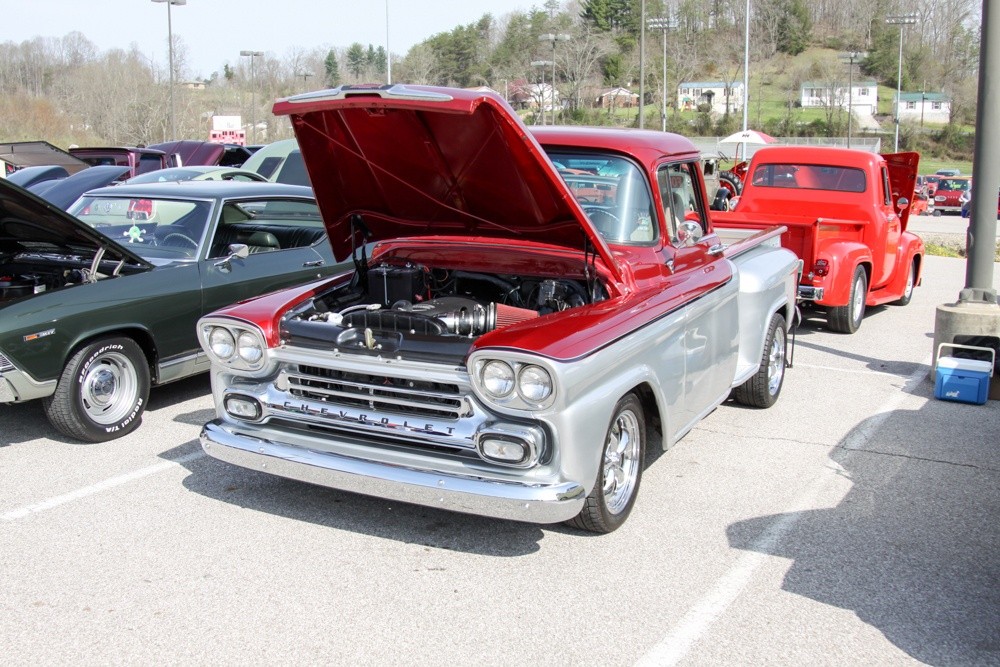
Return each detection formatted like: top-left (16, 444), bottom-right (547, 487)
top-left (663, 192), bottom-right (684, 223)
top-left (156, 225), bottom-right (194, 239)
top-left (941, 180), bottom-right (949, 189)
top-left (616, 175), bottom-right (650, 212)
top-left (961, 182), bottom-right (968, 190)
top-left (246, 232), bottom-right (280, 253)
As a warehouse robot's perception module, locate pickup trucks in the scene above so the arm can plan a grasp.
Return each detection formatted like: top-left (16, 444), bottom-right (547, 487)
top-left (683, 146), bottom-right (926, 335)
top-left (192, 85), bottom-right (818, 536)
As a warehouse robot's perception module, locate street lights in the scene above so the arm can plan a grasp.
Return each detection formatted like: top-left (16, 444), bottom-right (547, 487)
top-left (880, 12), bottom-right (921, 152)
top-left (151, 0), bottom-right (188, 137)
top-left (645, 15), bottom-right (679, 131)
top-left (837, 50), bottom-right (868, 149)
top-left (240, 50), bottom-right (265, 136)
top-left (538, 34), bottom-right (570, 126)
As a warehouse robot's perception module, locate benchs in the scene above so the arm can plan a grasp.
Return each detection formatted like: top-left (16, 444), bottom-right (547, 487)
top-left (207, 225), bottom-right (325, 258)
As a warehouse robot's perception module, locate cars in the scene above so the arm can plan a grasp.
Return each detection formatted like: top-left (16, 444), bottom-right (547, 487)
top-left (5, 141), bottom-right (315, 190)
top-left (911, 175), bottom-right (971, 217)
top-left (1, 169), bottom-right (339, 440)
